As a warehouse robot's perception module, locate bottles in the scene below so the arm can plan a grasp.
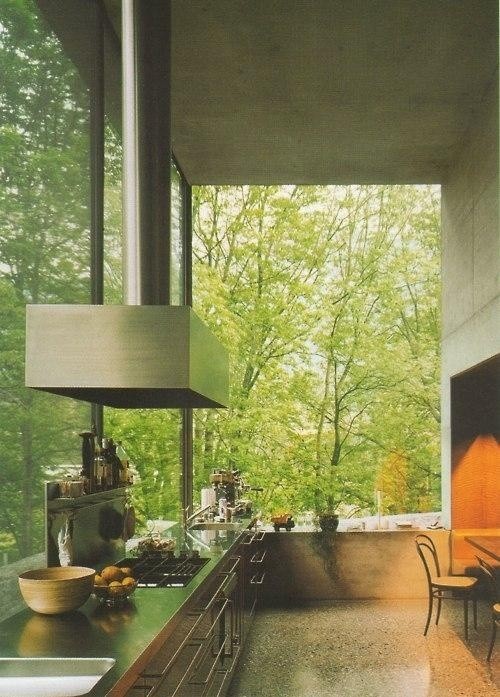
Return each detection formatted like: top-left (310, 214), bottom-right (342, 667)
top-left (79, 433), bottom-right (134, 494)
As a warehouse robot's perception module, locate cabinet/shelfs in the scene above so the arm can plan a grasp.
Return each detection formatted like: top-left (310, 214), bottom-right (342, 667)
top-left (1, 507), bottom-right (266, 697)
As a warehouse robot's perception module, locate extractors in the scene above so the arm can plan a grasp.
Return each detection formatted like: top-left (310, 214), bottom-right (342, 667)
top-left (25, 1), bottom-right (230, 410)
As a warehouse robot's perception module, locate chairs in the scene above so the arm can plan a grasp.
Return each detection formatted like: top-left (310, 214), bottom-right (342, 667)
top-left (411, 531), bottom-right (499, 662)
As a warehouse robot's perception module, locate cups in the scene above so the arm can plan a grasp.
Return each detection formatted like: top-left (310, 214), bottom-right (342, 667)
top-left (58, 481), bottom-right (83, 497)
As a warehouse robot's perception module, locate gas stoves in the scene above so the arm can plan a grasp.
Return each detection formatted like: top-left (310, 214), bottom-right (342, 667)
top-left (102, 556), bottom-right (210, 587)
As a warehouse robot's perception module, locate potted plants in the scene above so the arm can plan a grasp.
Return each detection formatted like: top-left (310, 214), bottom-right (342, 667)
top-left (297, 466), bottom-right (370, 532)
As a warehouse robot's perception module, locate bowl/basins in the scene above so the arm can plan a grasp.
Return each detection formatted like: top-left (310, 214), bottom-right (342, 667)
top-left (19, 566), bottom-right (137, 615)
